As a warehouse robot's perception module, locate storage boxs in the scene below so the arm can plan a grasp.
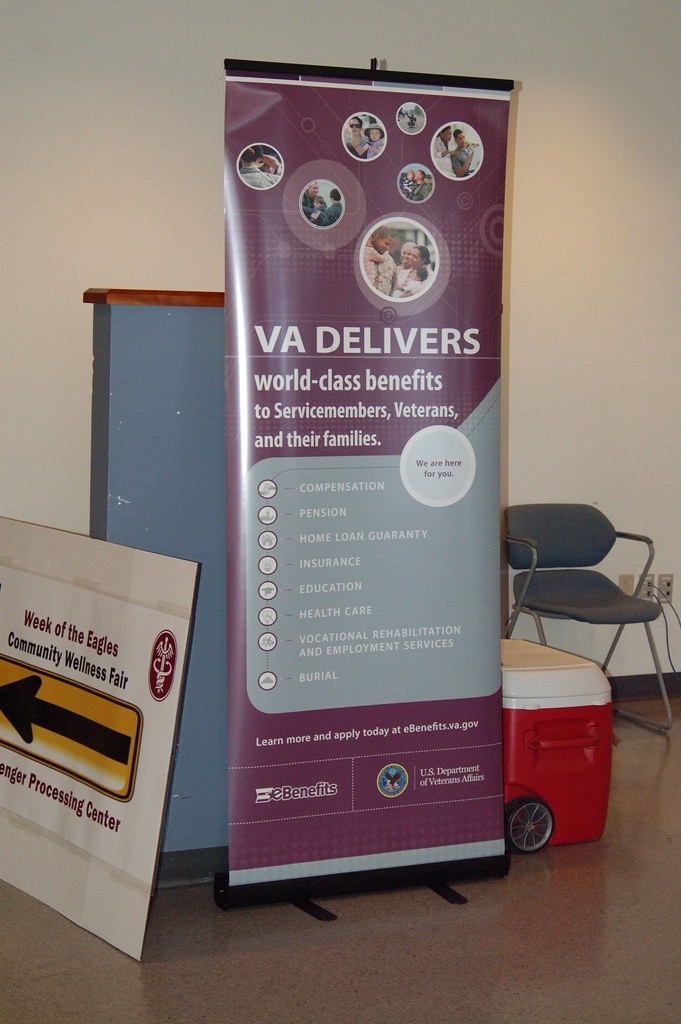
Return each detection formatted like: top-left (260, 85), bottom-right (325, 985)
top-left (499, 637), bottom-right (618, 852)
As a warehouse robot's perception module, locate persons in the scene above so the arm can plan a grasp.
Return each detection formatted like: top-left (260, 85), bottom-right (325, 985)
top-left (410, 170), bottom-right (433, 200)
top-left (312, 197), bottom-right (329, 227)
top-left (371, 223), bottom-right (399, 296)
top-left (451, 129), bottom-right (476, 178)
top-left (241, 145), bottom-right (283, 188)
top-left (346, 116), bottom-right (368, 158)
top-left (391, 245), bottom-right (434, 298)
top-left (435, 125), bottom-right (461, 157)
top-left (364, 235), bottom-right (389, 284)
top-left (394, 267), bottom-right (428, 298)
top-left (303, 182), bottom-right (327, 224)
top-left (257, 148), bottom-right (282, 176)
top-left (396, 241), bottom-right (417, 273)
top-left (324, 188), bottom-right (342, 226)
top-left (403, 170), bottom-right (416, 198)
top-left (364, 123), bottom-right (384, 159)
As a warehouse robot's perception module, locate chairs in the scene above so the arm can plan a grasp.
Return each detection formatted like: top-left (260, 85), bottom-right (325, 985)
top-left (500, 502), bottom-right (673, 733)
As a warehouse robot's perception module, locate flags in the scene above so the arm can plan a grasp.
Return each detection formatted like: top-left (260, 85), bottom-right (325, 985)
top-left (355, 140), bottom-right (370, 156)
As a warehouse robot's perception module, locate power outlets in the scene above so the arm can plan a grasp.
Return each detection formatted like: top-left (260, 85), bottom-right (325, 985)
top-left (638, 573), bottom-right (656, 603)
top-left (657, 574), bottom-right (673, 603)
